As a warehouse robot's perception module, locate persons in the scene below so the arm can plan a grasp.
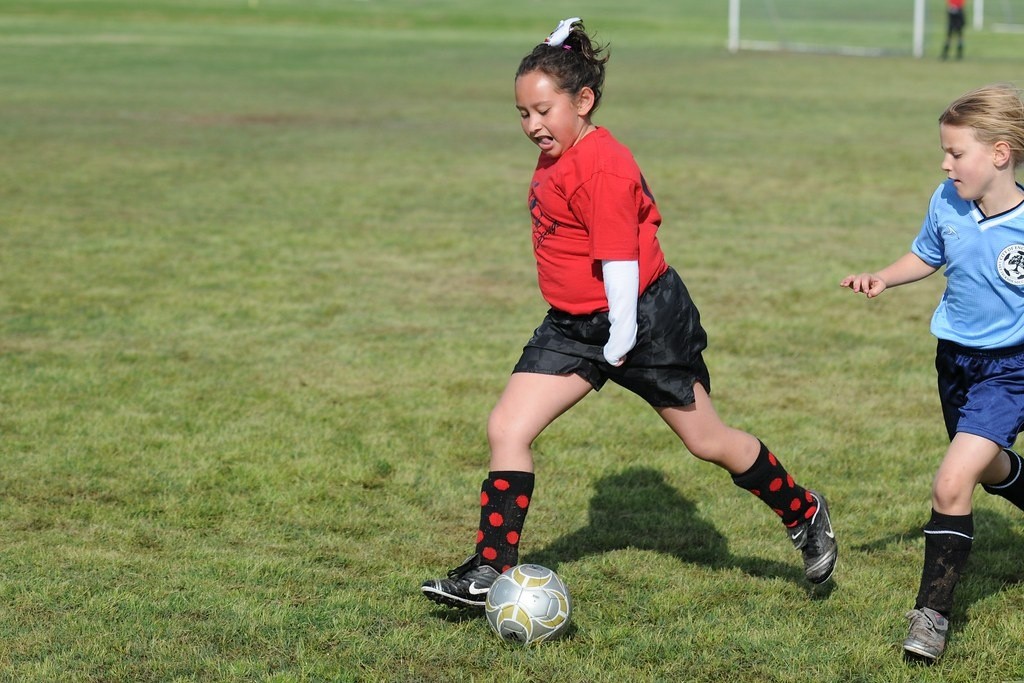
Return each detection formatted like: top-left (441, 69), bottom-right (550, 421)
top-left (840, 85), bottom-right (1024, 660)
top-left (421, 17), bottom-right (837, 610)
top-left (941, 1), bottom-right (967, 61)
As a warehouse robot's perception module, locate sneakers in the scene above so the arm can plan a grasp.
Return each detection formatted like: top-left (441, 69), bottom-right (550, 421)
top-left (422, 554), bottom-right (501, 610)
top-left (786, 492), bottom-right (838, 585)
top-left (904, 607), bottom-right (949, 660)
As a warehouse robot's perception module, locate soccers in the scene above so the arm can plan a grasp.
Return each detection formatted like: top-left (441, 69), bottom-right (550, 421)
top-left (486, 565), bottom-right (572, 646)
